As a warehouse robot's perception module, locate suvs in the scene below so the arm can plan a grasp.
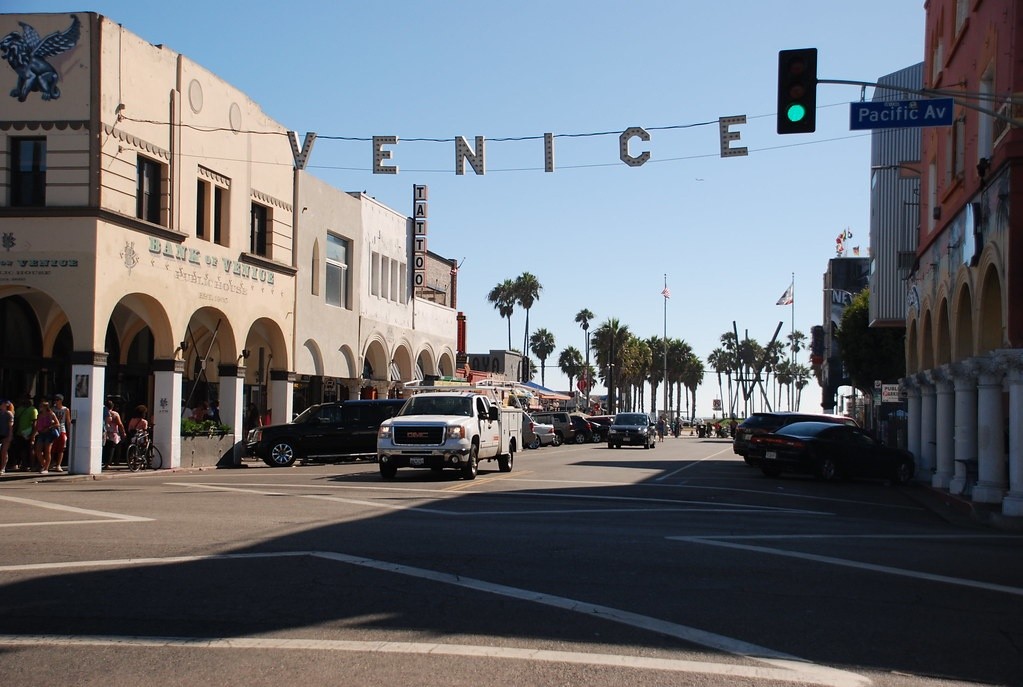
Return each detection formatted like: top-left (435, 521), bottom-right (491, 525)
top-left (570, 415), bottom-right (592, 444)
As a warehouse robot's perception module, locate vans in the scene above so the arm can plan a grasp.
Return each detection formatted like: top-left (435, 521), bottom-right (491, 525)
top-left (522, 411), bottom-right (534, 449)
top-left (248, 398), bottom-right (437, 473)
top-left (531, 412), bottom-right (575, 446)
top-left (732, 412), bottom-right (861, 470)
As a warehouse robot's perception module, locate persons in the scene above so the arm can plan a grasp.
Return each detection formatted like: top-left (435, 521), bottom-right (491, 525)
top-left (103, 399), bottom-right (154, 469)
top-left (706, 421), bottom-right (720, 438)
top-left (727, 422), bottom-right (730, 429)
top-left (657, 416), bottom-right (664, 442)
top-left (181, 398), bottom-right (221, 426)
top-left (245, 402), bottom-right (263, 427)
top-left (0, 393), bottom-right (71, 474)
top-left (422, 399), bottom-right (433, 414)
top-left (462, 400), bottom-right (479, 416)
top-left (730, 415), bottom-right (738, 440)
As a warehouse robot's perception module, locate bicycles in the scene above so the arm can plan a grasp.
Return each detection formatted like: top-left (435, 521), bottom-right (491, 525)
top-left (126, 423), bottom-right (163, 473)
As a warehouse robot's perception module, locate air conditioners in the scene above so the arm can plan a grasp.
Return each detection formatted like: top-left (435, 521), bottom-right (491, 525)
top-left (896, 251), bottom-right (917, 269)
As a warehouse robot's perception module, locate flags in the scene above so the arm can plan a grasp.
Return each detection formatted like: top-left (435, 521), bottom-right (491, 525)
top-left (775, 283), bottom-right (793, 305)
top-left (660, 287), bottom-right (670, 298)
top-left (835, 228), bottom-right (860, 257)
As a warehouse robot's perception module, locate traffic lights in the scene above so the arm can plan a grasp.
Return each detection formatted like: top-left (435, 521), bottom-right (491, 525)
top-left (776, 47), bottom-right (818, 135)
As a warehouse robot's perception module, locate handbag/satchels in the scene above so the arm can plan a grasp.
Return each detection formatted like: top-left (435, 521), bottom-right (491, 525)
top-left (48, 427), bottom-right (61, 440)
top-left (128, 428), bottom-right (137, 438)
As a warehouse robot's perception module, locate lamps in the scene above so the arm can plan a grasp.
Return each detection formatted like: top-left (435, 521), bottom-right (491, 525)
top-left (977, 157), bottom-right (992, 179)
top-left (177, 341), bottom-right (191, 352)
top-left (388, 359), bottom-right (396, 365)
top-left (239, 350), bottom-right (251, 359)
top-left (358, 378), bottom-right (370, 389)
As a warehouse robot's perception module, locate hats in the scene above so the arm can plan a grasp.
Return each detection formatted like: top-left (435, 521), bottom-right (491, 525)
top-left (54, 394), bottom-right (62, 399)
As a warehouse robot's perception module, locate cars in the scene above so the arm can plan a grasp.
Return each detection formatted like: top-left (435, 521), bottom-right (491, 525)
top-left (586, 415), bottom-right (617, 442)
top-left (528, 416), bottom-right (556, 450)
top-left (746, 422), bottom-right (916, 483)
top-left (607, 412), bottom-right (657, 450)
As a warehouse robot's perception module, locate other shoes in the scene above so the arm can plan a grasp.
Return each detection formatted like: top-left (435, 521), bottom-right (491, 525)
top-left (56, 466), bottom-right (62, 471)
top-left (40, 470), bottom-right (48, 473)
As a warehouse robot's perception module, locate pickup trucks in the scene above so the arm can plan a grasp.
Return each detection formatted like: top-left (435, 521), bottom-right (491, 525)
top-left (376, 392), bottom-right (524, 480)
